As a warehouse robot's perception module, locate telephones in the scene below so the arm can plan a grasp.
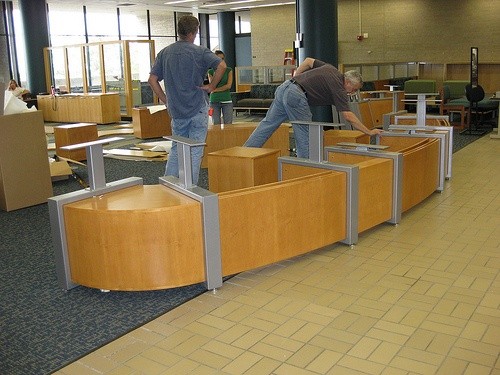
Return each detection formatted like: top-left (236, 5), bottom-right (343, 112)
top-left (52, 88), bottom-right (60, 94)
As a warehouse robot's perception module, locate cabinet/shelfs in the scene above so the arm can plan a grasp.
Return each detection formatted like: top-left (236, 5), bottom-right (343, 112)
top-left (324, 130), bottom-right (370, 145)
top-left (207, 145), bottom-right (280, 193)
top-left (36, 91), bottom-right (120, 125)
top-left (132, 107), bottom-right (172, 139)
top-left (54, 122), bottom-right (98, 162)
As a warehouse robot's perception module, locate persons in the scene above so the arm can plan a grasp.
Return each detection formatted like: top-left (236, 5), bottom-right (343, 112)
top-left (4, 79), bottom-right (31, 100)
top-left (243, 57), bottom-right (386, 161)
top-left (208, 50), bottom-right (234, 125)
top-left (148, 16), bottom-right (227, 185)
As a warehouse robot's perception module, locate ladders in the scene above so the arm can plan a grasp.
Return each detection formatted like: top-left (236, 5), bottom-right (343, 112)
top-left (284, 49), bottom-right (294, 80)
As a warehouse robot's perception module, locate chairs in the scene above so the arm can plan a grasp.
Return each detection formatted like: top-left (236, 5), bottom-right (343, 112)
top-left (439, 87), bottom-right (470, 131)
top-left (389, 76), bottom-right (470, 100)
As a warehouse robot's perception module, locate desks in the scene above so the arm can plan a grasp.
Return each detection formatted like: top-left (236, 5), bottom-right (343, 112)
top-left (446, 96), bottom-right (500, 128)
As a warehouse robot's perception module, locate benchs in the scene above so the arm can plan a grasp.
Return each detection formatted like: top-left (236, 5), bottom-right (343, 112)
top-left (230, 84), bottom-right (282, 117)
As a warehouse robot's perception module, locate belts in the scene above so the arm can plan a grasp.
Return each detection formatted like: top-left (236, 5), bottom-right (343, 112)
top-left (290, 78), bottom-right (308, 97)
